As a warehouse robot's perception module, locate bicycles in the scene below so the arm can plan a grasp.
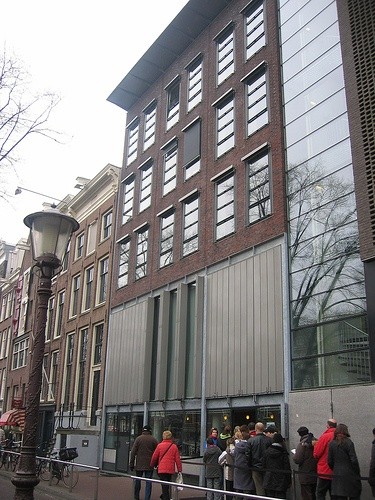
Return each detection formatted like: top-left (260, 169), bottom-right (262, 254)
top-left (0, 440), bottom-right (22, 472)
top-left (37, 448), bottom-right (80, 488)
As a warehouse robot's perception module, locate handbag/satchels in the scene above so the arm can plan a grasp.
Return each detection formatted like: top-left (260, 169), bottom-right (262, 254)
top-left (175, 472), bottom-right (183, 491)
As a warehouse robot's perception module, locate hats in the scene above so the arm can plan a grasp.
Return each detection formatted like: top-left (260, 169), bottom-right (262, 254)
top-left (163, 431), bottom-right (172, 439)
top-left (144, 426), bottom-right (152, 431)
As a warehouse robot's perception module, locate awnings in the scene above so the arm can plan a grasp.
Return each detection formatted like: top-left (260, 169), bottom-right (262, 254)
top-left (0, 409), bottom-right (25, 431)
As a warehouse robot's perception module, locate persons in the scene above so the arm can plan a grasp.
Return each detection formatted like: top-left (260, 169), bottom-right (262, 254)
top-left (368, 428), bottom-right (375, 500)
top-left (262, 433), bottom-right (291, 499)
top-left (203, 422), bottom-right (278, 500)
top-left (128, 425), bottom-right (157, 500)
top-left (293, 426), bottom-right (318, 500)
top-left (327, 424), bottom-right (362, 500)
top-left (0, 427), bottom-right (15, 447)
top-left (150, 432), bottom-right (182, 500)
top-left (312, 419), bottom-right (337, 500)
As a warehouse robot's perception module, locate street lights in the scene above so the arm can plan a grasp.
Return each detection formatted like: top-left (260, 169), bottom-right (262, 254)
top-left (10, 204), bottom-right (80, 500)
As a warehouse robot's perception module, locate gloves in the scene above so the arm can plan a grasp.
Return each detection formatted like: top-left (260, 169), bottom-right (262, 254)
top-left (130, 467), bottom-right (134, 470)
